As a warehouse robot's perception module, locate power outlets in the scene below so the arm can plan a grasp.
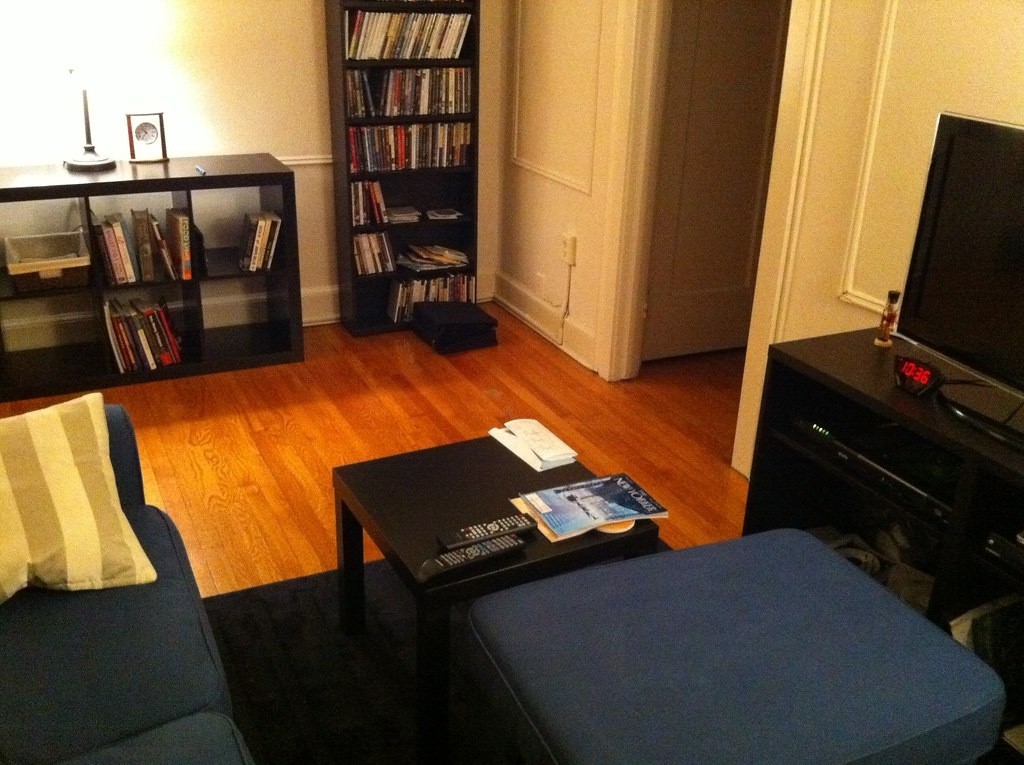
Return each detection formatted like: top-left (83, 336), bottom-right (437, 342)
top-left (559, 233), bottom-right (576, 265)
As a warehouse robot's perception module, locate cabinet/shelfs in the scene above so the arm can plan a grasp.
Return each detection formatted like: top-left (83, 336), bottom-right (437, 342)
top-left (742, 326), bottom-right (1024, 631)
top-left (325, 0), bottom-right (481, 339)
top-left (0, 152), bottom-right (305, 403)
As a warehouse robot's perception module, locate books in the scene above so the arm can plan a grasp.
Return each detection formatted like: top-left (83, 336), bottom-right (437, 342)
top-left (386, 273), bottom-right (475, 323)
top-left (345, 68), bottom-right (471, 118)
top-left (384, 205), bottom-right (421, 224)
top-left (345, 10), bottom-right (471, 58)
top-left (354, 231), bottom-right (396, 275)
top-left (522, 471), bottom-right (669, 538)
top-left (238, 211), bottom-right (282, 272)
top-left (103, 298), bottom-right (182, 373)
top-left (349, 180), bottom-right (388, 226)
top-left (349, 122), bottom-right (470, 173)
top-left (89, 208), bottom-right (193, 285)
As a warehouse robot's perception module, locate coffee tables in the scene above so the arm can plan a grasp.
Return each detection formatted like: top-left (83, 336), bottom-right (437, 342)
top-left (333, 434), bottom-right (658, 765)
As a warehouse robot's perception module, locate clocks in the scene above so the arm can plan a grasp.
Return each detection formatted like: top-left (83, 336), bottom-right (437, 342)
top-left (125, 111), bottom-right (171, 165)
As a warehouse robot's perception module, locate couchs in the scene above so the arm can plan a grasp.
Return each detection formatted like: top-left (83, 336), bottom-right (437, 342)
top-left (0, 404), bottom-right (255, 765)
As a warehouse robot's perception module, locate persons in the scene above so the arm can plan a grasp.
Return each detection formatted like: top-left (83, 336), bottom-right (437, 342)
top-left (879, 290), bottom-right (900, 342)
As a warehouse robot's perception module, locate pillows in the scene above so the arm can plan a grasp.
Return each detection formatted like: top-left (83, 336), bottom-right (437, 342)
top-left (0, 392), bottom-right (157, 605)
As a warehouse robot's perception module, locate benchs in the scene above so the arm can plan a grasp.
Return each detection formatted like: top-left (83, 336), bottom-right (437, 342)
top-left (464, 527), bottom-right (1007, 765)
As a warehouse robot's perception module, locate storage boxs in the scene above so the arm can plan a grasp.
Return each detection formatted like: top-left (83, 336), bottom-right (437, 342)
top-left (414, 302), bottom-right (499, 355)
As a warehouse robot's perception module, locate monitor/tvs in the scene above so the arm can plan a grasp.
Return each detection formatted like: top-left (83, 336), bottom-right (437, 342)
top-left (896, 109), bottom-right (1024, 452)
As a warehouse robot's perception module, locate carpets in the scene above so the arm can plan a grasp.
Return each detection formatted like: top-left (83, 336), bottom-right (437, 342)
top-left (203, 536), bottom-right (674, 765)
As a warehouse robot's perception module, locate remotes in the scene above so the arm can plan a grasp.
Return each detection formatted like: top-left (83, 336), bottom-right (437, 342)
top-left (416, 533), bottom-right (527, 585)
top-left (434, 513), bottom-right (539, 552)
